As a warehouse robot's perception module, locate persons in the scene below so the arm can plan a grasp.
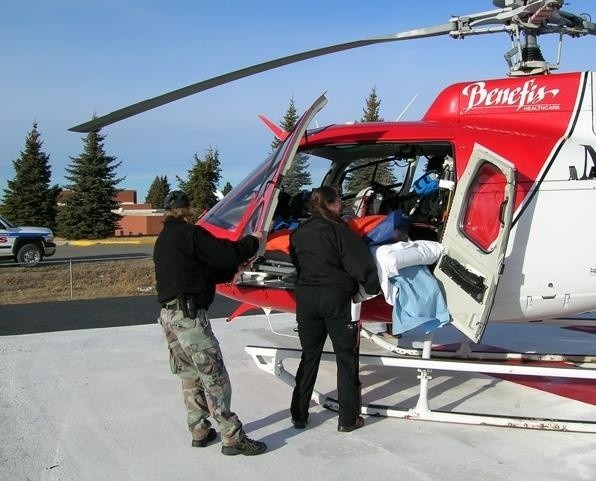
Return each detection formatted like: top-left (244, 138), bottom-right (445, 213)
top-left (290, 185), bottom-right (379, 432)
top-left (153, 189), bottom-right (268, 455)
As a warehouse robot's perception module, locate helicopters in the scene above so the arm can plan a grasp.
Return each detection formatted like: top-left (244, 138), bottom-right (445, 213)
top-left (66, 1), bottom-right (596, 435)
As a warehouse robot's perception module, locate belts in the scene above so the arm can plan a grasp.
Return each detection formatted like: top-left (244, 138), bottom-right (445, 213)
top-left (162, 303), bottom-right (182, 309)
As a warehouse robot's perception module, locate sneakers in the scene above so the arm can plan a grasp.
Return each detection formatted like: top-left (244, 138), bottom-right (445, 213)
top-left (292, 419), bottom-right (306, 428)
top-left (192, 428), bottom-right (217, 446)
top-left (222, 437), bottom-right (267, 455)
top-left (338, 416), bottom-right (364, 432)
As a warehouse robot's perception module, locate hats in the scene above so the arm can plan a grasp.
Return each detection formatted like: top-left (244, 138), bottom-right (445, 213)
top-left (163, 191), bottom-right (189, 209)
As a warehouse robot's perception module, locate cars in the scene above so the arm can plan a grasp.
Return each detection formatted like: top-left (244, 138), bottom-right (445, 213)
top-left (0, 215), bottom-right (56, 269)
